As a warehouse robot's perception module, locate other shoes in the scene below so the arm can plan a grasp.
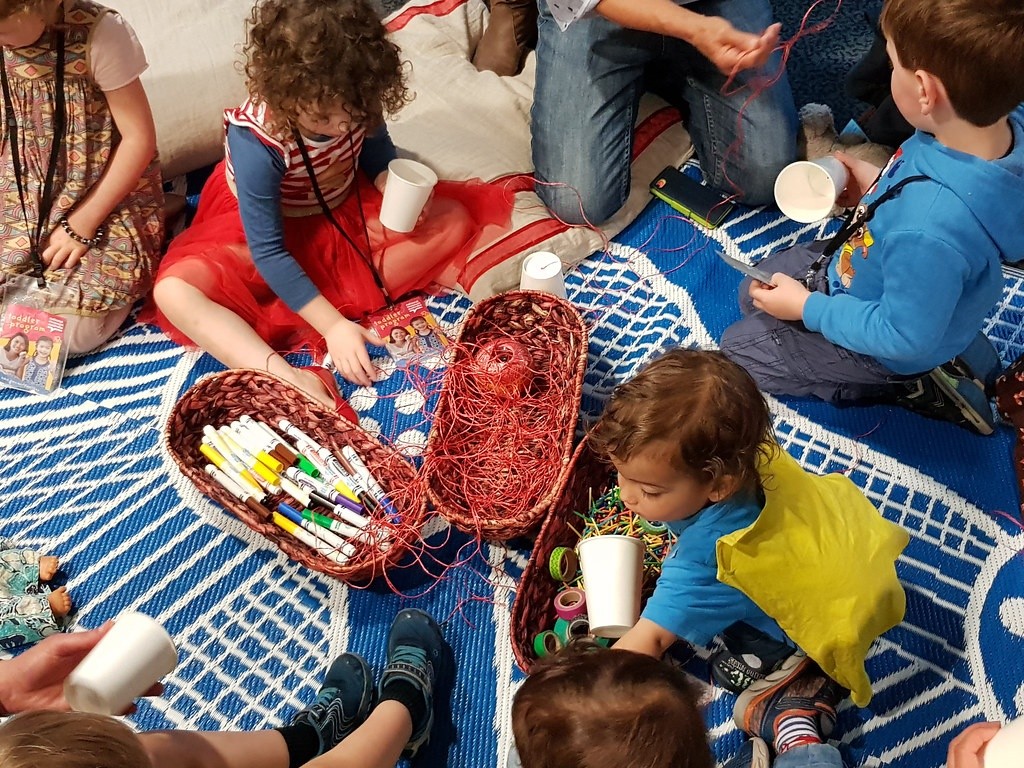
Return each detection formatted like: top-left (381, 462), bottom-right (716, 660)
top-left (302, 366), bottom-right (360, 425)
top-left (723, 737), bottom-right (769, 768)
top-left (710, 650), bottom-right (770, 693)
top-left (473, 0), bottom-right (536, 76)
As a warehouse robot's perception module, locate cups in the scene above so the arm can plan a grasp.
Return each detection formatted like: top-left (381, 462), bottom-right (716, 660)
top-left (773, 156), bottom-right (850, 223)
top-left (576, 534), bottom-right (646, 638)
top-left (520, 252), bottom-right (567, 299)
top-left (379, 158), bottom-right (438, 232)
top-left (62, 610), bottom-right (179, 719)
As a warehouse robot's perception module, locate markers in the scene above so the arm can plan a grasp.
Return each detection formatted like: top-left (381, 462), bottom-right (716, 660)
top-left (277, 502), bottom-right (357, 558)
top-left (200, 415), bottom-right (385, 520)
top-left (342, 445), bottom-right (401, 524)
top-left (301, 508), bottom-right (392, 551)
top-left (272, 511), bottom-right (349, 566)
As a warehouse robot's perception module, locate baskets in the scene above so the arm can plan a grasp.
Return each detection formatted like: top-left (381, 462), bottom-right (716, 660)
top-left (424, 289), bottom-right (588, 541)
top-left (509, 423), bottom-right (673, 673)
top-left (165, 368), bottom-right (426, 581)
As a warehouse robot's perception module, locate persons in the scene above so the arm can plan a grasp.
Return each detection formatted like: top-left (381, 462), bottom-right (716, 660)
top-left (0, 0), bottom-right (171, 352)
top-left (386, 327), bottom-right (414, 353)
top-left (607, 348), bottom-right (910, 709)
top-left (533, 0), bottom-right (800, 227)
top-left (24, 336), bottom-right (55, 386)
top-left (0, 607), bottom-right (447, 767)
top-left (0, 332), bottom-right (29, 379)
top-left (410, 316), bottom-right (442, 352)
top-left (155, 0), bottom-right (514, 433)
top-left (723, 0), bottom-right (1024, 433)
top-left (0, 550), bottom-right (70, 651)
top-left (514, 650), bottom-right (1002, 768)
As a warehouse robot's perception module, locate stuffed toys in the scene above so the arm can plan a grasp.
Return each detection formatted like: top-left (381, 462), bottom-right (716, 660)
top-left (797, 103), bottom-right (889, 170)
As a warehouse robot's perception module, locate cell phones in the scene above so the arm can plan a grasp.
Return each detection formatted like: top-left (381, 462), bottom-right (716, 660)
top-left (649, 166), bottom-right (733, 230)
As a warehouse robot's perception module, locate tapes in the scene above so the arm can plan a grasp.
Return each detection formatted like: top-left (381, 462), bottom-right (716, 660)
top-left (553, 614), bottom-right (611, 653)
top-left (534, 630), bottom-right (562, 658)
top-left (554, 588), bottom-right (585, 620)
top-left (549, 547), bottom-right (577, 582)
top-left (640, 516), bottom-right (668, 534)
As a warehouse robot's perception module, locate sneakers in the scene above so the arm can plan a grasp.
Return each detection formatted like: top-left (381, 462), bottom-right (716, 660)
top-left (293, 651), bottom-right (372, 757)
top-left (378, 608), bottom-right (446, 758)
top-left (949, 330), bottom-right (1002, 389)
top-left (733, 650), bottom-right (850, 744)
top-left (893, 364), bottom-right (994, 435)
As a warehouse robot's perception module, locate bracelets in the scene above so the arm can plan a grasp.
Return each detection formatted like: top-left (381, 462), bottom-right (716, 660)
top-left (60, 209), bottom-right (103, 245)
